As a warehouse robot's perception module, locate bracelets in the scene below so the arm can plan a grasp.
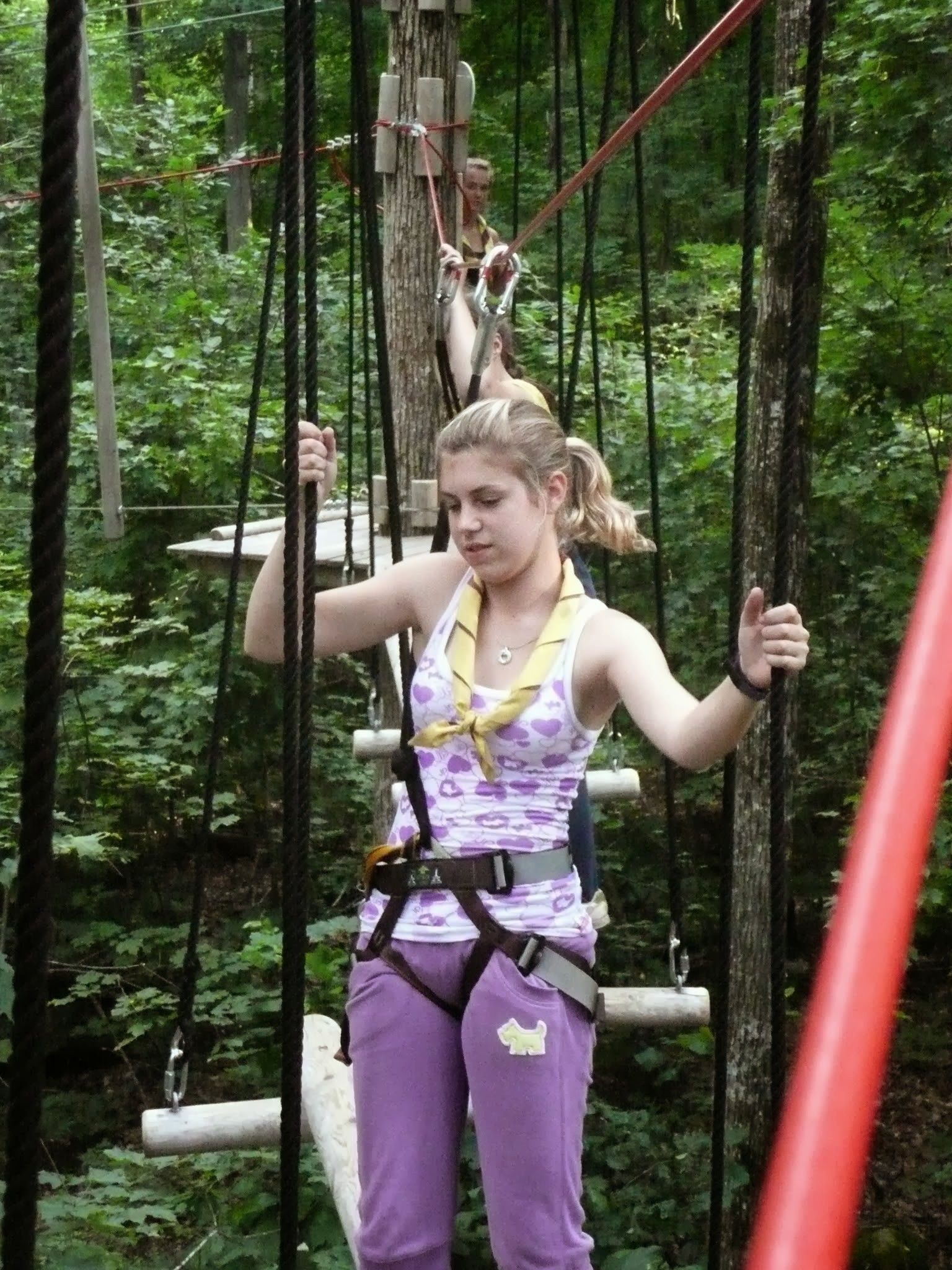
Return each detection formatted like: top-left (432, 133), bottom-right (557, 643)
top-left (725, 651), bottom-right (772, 701)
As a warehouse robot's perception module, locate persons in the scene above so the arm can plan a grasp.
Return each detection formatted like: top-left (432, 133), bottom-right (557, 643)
top-left (462, 158), bottom-right (501, 287)
top-left (437, 244), bottom-right (610, 932)
top-left (243, 399), bottom-right (810, 1270)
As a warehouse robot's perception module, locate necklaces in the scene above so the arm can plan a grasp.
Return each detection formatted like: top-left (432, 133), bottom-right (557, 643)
top-left (481, 603), bottom-right (541, 665)
